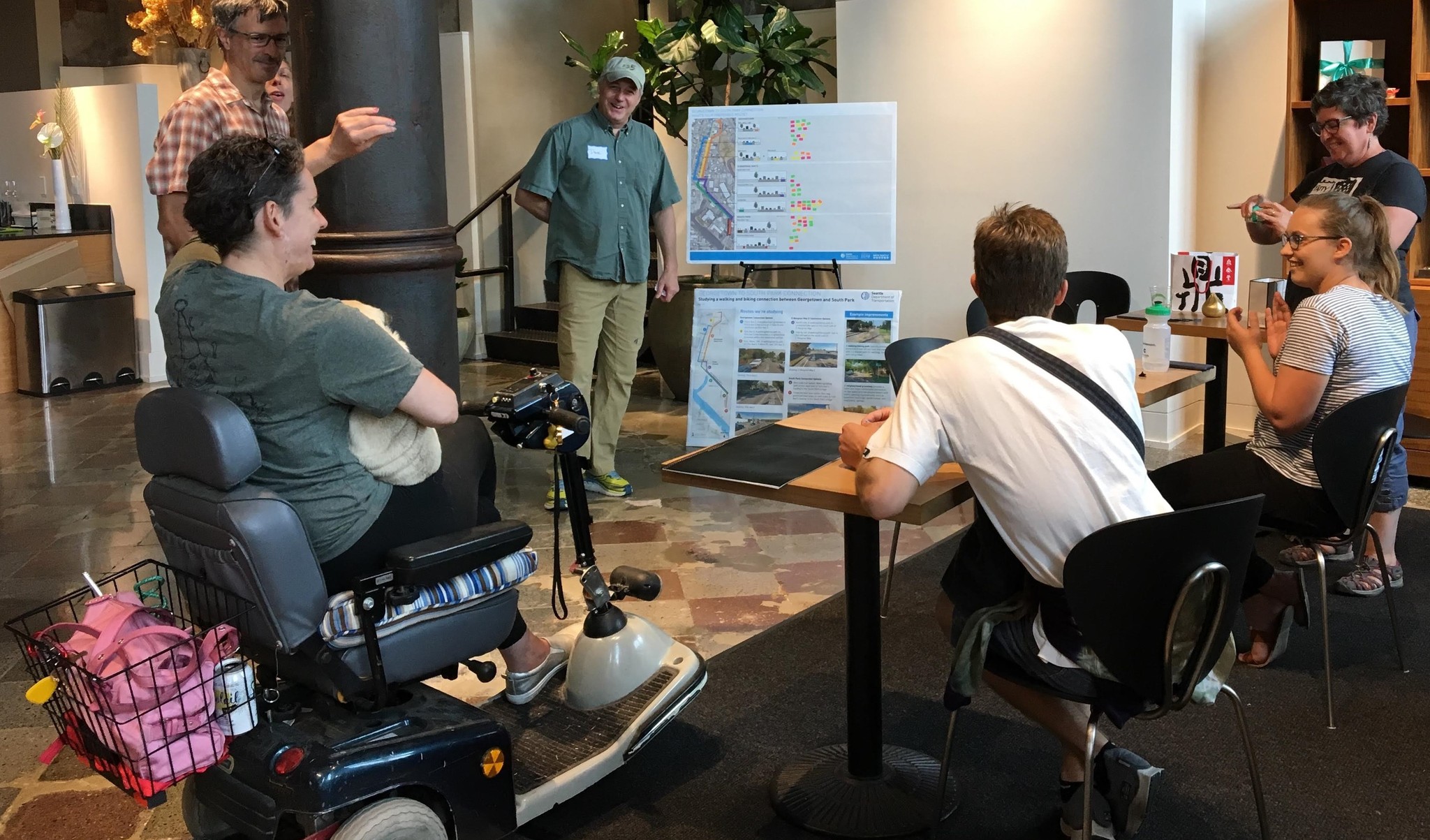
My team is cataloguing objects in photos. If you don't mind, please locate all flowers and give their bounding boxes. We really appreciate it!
[30,74,81,160]
[125,0,220,58]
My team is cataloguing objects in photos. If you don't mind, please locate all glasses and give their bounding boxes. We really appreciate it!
[248,137,286,198]
[222,25,291,47]
[1310,115,1354,136]
[1281,233,1342,251]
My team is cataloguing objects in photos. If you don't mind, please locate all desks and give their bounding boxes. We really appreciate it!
[1104,308,1268,454]
[1132,357,1217,408]
[662,408,973,840]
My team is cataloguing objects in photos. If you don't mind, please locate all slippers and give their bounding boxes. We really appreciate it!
[1238,604,1295,668]
[1289,567,1310,629]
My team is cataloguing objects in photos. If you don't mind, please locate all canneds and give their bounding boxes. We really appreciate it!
[213,657,257,736]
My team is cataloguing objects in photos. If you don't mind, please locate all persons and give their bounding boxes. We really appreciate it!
[1149,190,1417,669]
[515,56,680,512]
[1227,73,1426,597]
[145,0,302,296]
[156,105,586,705]
[839,200,1238,839]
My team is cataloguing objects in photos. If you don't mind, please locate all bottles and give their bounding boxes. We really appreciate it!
[1141,301,1171,373]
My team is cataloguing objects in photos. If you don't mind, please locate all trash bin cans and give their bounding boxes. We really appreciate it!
[13,281,143,397]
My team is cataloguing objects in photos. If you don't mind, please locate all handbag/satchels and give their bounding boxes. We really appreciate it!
[1247,277,1287,329]
[937,512,1040,611]
[34,593,240,783]
[1170,251,1240,314]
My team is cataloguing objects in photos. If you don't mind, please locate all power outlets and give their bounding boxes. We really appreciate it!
[39,176,47,195]
[71,176,79,195]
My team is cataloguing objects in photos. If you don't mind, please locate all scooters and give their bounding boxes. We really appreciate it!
[0,366,708,840]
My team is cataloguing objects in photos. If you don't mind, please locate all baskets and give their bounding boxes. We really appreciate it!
[4,558,288,810]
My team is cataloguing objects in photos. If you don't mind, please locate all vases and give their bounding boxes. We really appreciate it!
[52,158,72,230]
[174,46,212,94]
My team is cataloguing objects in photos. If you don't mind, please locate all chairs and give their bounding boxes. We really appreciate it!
[880,271,1414,840]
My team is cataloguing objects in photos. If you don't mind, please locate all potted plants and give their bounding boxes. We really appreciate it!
[558,0,836,403]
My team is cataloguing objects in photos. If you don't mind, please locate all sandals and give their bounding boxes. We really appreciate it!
[1334,555,1404,597]
[1279,534,1355,566]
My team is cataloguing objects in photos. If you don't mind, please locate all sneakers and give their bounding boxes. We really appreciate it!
[501,621,585,705]
[544,479,568,511]
[1103,747,1167,840]
[1059,783,1116,840]
[583,470,633,497]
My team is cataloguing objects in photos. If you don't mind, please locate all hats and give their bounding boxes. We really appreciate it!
[600,56,646,91]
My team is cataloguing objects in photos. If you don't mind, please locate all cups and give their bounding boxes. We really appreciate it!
[1149,285,1176,312]
